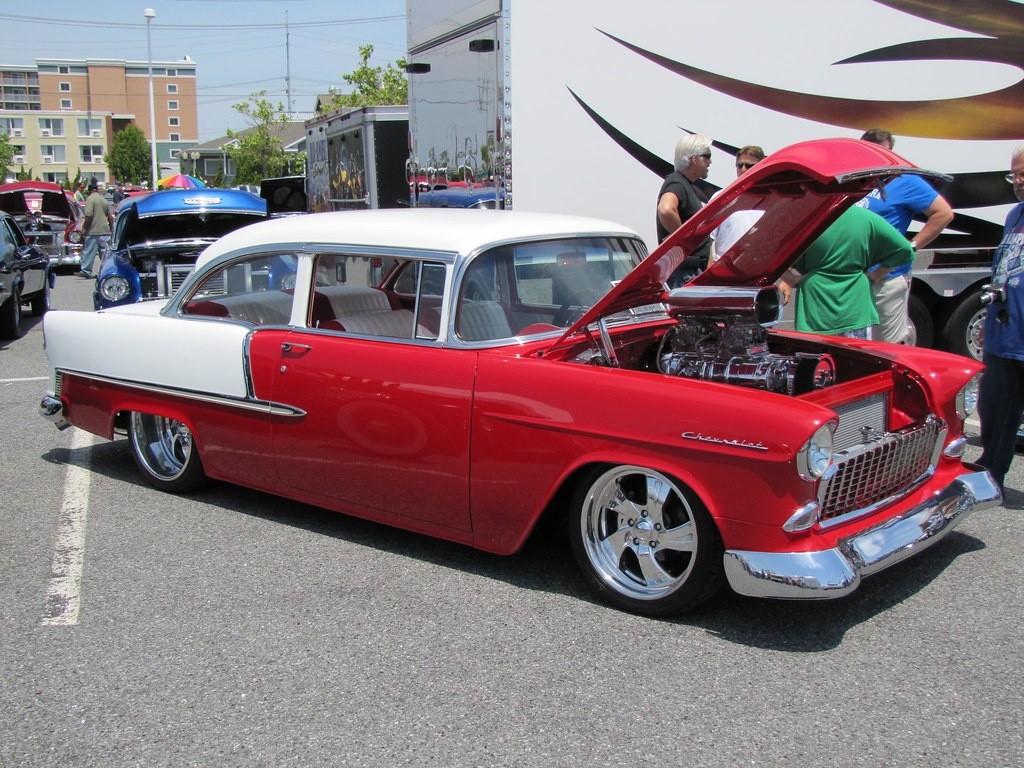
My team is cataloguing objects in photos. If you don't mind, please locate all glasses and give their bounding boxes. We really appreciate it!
[1004,170,1024,184]
[735,162,755,168]
[689,153,712,159]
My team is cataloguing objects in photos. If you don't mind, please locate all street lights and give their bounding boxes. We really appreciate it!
[144,6,159,191]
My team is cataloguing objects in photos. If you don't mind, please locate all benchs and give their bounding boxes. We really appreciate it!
[318,300,520,340]
[187,284,402,324]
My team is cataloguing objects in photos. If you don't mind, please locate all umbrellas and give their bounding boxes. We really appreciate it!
[154,174,206,189]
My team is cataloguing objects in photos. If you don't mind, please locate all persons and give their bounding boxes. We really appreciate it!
[113,184,125,205]
[855,129,955,343]
[776,204,915,338]
[707,146,791,306]
[90,176,98,184]
[74,184,113,278]
[657,135,713,290]
[74,183,85,201]
[974,146,1024,492]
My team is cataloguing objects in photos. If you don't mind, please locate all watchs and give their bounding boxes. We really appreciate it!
[910,239,917,251]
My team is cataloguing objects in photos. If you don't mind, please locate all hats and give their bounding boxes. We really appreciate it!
[77,182,86,188]
[118,184,124,186]
[88,184,98,191]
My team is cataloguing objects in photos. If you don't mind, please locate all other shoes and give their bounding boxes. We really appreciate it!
[74,272,92,279]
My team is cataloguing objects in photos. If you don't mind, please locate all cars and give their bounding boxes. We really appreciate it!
[0,211,57,338]
[36,135,1003,616]
[0,181,83,268]
[91,187,298,312]
